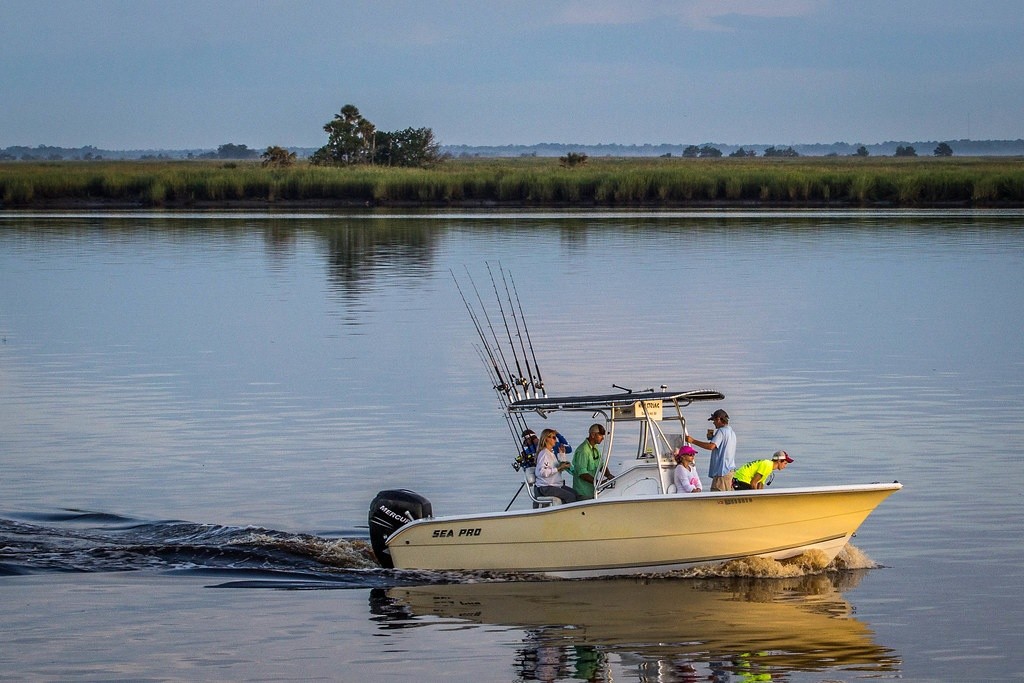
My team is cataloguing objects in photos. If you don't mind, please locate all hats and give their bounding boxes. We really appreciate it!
[708,409,728,421]
[679,446,698,456]
[772,450,794,463]
[589,424,610,435]
[522,434,535,447]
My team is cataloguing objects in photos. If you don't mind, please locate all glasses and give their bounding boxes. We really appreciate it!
[683,454,695,456]
[549,436,556,440]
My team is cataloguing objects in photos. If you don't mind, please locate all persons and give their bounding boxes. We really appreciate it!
[732,450,795,490]
[534,428,579,503]
[674,446,702,493]
[572,423,616,502]
[685,409,737,491]
[520,429,572,509]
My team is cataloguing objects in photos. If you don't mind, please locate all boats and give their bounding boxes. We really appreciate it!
[360,253,907,574]
